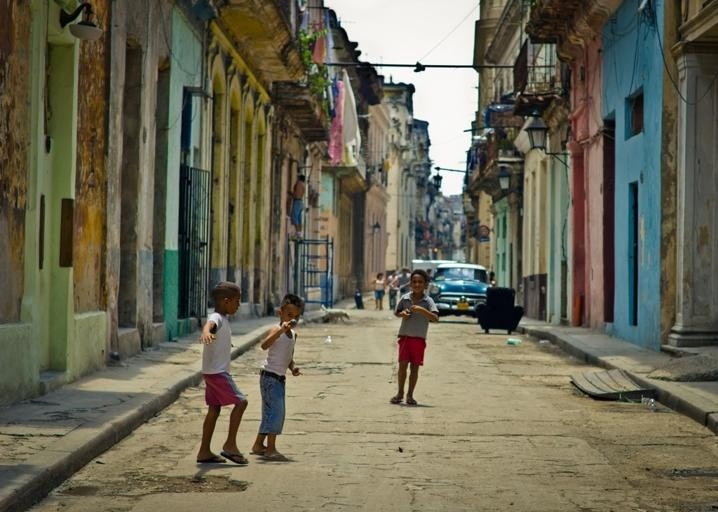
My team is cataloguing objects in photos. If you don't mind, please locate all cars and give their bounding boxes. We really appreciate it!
[427,263,497,324]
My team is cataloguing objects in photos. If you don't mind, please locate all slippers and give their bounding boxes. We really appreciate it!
[391,397,417,405]
[197,447,288,465]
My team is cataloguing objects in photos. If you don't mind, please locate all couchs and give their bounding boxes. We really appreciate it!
[471,285,527,337]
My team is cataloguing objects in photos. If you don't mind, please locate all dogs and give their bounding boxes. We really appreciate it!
[320,304,351,324]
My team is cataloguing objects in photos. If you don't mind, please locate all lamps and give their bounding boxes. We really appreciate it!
[433,166,477,193]
[496,164,523,196]
[57,1,103,42]
[522,105,573,169]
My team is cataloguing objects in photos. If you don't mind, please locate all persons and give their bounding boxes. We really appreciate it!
[487,269,497,286]
[196,281,250,466]
[369,267,434,309]
[249,294,304,462]
[289,174,305,239]
[388,271,439,404]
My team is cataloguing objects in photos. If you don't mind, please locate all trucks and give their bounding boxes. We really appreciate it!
[411,258,458,282]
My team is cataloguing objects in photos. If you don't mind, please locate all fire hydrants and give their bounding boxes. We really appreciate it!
[352,289,364,309]
